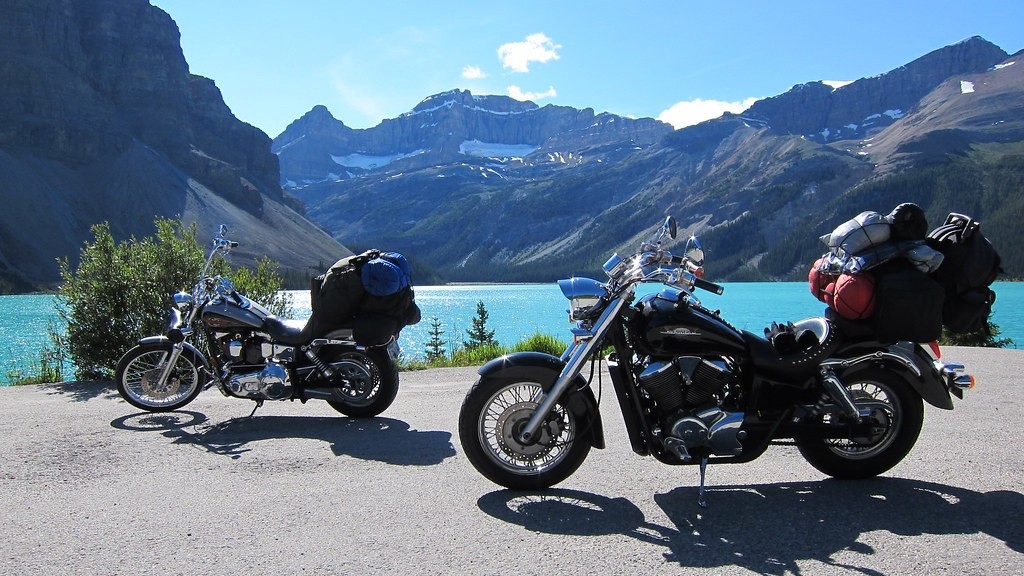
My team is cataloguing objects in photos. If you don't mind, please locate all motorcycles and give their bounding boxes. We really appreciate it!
[457,216,975,508]
[115,223,404,419]
